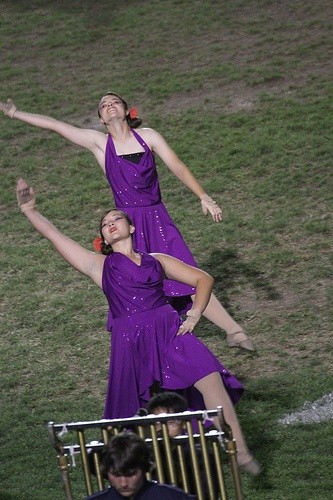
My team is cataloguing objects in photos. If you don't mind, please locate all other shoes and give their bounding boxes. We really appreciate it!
[229,453,262,475]
[226,331,256,351]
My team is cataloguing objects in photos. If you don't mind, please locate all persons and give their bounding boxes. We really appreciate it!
[16,177,265,481]
[82,431,199,500]
[87,391,224,500]
[0,91,260,358]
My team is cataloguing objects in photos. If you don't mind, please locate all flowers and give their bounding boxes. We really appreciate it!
[130,107,137,118]
[93,237,102,252]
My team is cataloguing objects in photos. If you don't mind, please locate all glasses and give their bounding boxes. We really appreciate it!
[153,419,179,430]
